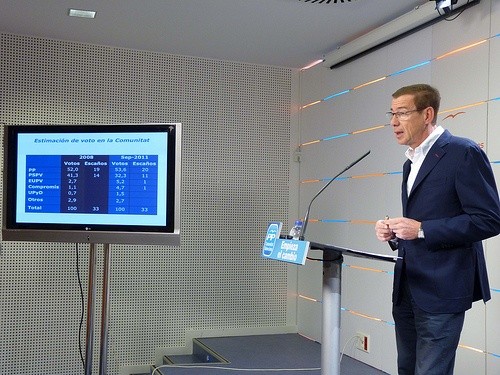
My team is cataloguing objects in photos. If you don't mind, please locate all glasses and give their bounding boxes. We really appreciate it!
[385,109,422,120]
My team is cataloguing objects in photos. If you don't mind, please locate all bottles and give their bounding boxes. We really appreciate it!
[289,220,303,240]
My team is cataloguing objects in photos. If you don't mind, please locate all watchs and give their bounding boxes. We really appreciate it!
[417,224,426,239]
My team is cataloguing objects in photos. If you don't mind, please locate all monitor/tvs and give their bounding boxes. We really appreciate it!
[0,122,183,247]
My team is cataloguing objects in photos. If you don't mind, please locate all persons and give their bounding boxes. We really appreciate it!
[372,82,500,375]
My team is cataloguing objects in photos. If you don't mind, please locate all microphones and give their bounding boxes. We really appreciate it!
[298,150,372,241]
